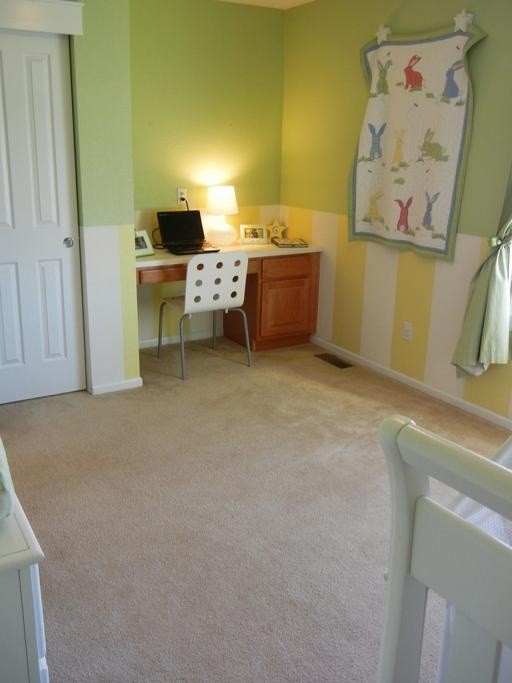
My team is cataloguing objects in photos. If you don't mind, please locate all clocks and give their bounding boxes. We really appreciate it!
[267,219,286,240]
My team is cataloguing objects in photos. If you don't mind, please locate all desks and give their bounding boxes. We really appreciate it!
[134,241,323,352]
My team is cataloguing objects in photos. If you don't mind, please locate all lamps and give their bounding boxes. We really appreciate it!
[204,184,240,247]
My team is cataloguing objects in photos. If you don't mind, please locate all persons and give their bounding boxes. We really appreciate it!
[251,229,258,238]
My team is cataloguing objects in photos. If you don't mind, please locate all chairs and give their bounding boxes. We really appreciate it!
[375,413,511,681]
[153,251,253,379]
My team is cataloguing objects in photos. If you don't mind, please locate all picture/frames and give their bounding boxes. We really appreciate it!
[135,228,155,258]
[240,224,268,244]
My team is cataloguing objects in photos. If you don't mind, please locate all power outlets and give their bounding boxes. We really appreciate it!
[177,188,187,205]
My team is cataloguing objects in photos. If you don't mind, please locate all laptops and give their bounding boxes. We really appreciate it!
[156,210,220,255]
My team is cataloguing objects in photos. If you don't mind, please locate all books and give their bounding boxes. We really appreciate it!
[271,237,308,248]
